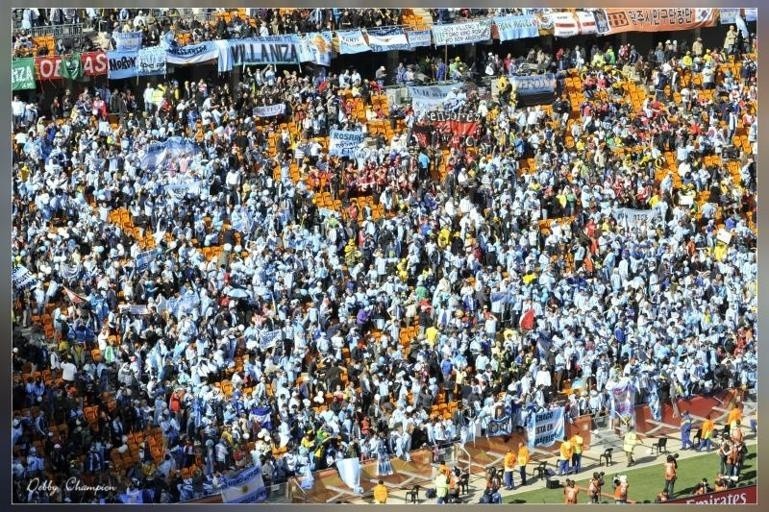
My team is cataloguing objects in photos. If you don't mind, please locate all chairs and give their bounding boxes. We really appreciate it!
[13,6,761,505]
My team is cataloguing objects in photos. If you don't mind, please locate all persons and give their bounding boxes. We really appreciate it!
[10,6,756,504]
